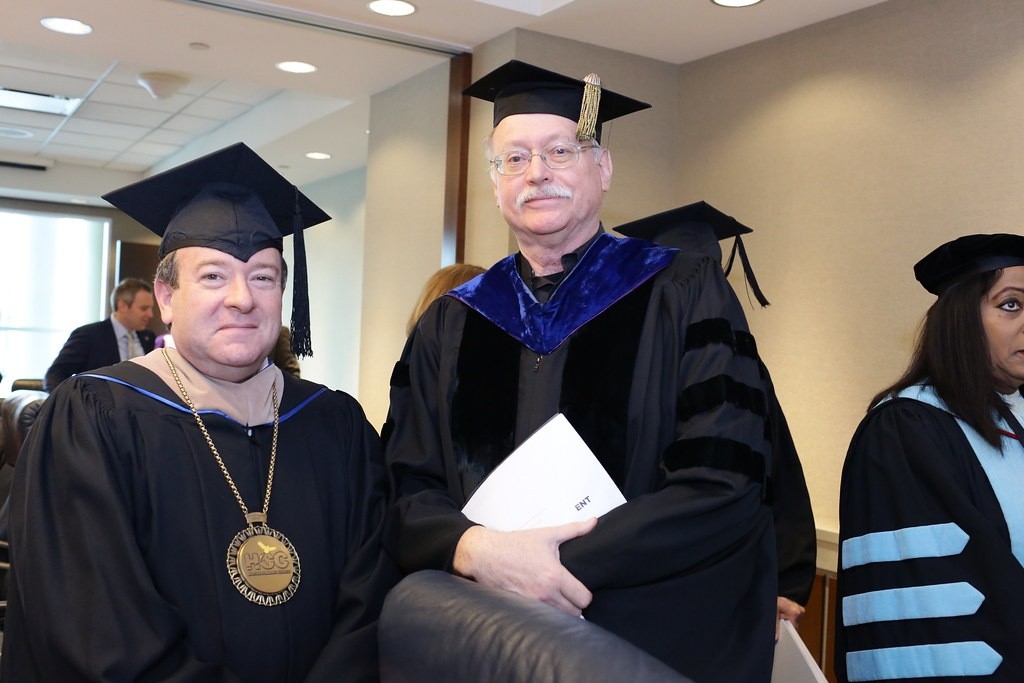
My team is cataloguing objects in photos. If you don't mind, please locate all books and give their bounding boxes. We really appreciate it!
[772,617,829,683]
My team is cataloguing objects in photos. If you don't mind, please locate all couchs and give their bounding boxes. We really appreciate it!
[0,390,48,620]
[377,570,693,683]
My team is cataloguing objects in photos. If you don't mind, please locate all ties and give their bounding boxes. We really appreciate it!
[123,334,137,359]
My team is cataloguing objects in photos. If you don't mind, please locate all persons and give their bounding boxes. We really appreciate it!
[384,59,819,683]
[0,139,383,683]
[833,232,1024,683]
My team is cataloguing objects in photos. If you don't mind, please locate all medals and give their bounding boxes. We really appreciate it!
[226,525,302,607]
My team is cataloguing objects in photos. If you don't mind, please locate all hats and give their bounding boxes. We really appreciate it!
[612,201,771,311]
[461,60,652,143]
[102,141,333,360]
[913,232,1024,296]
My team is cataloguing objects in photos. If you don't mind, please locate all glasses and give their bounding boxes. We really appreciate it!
[488,143,600,175]
